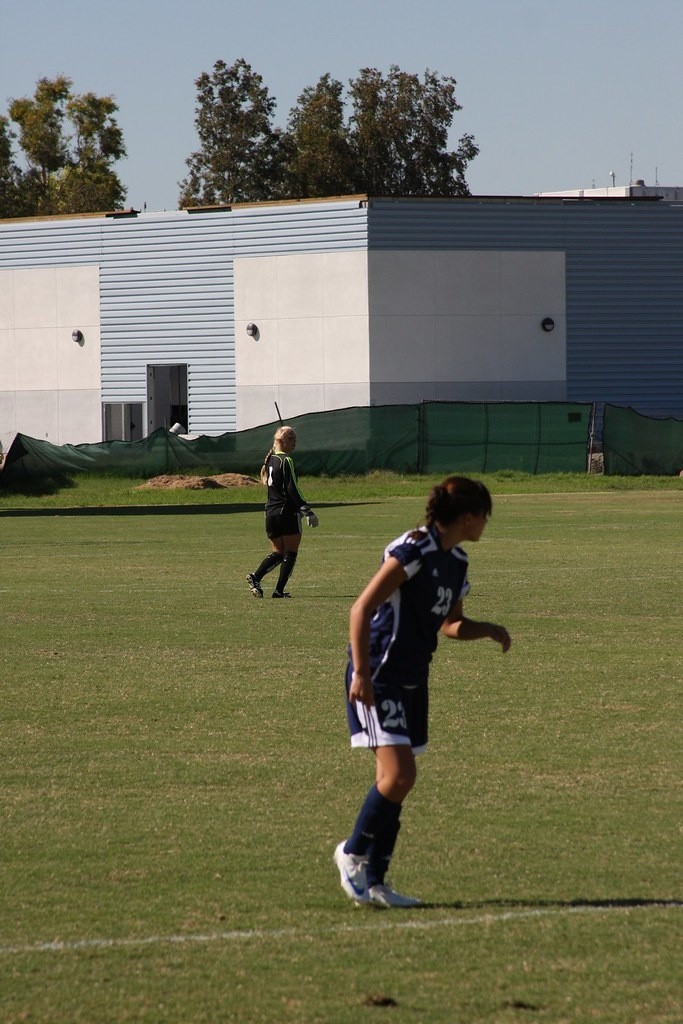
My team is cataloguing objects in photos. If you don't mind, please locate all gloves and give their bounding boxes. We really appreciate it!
[306,514,319,527]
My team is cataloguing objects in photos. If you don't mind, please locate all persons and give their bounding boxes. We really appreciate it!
[246,426,319,598]
[334,477,511,906]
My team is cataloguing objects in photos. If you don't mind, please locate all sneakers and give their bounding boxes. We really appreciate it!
[246,573,264,598]
[333,839,370,904]
[271,589,291,598]
[366,885,421,908]
